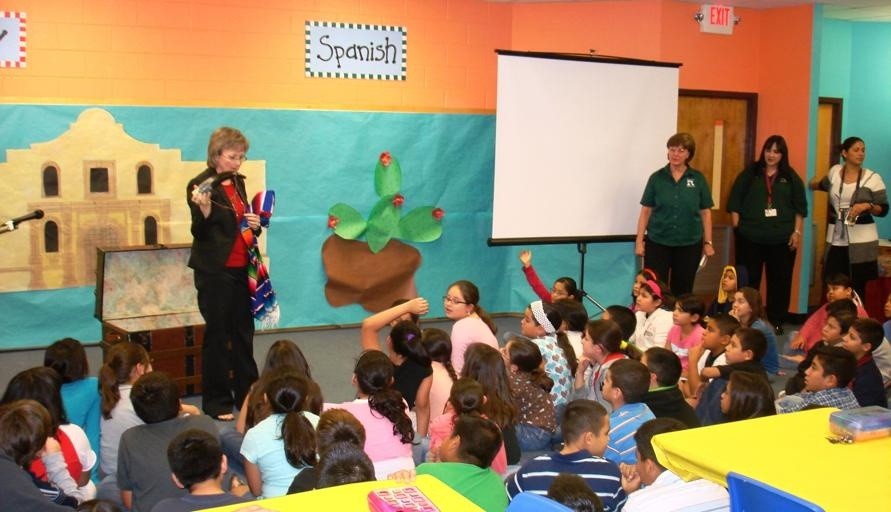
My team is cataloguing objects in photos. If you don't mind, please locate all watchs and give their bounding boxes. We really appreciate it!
[702,241,712,245]
[868,201,874,211]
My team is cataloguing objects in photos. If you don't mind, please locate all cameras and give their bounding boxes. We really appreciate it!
[843,212,859,226]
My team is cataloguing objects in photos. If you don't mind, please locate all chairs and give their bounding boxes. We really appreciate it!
[504,492,574,511]
[721,466,828,512]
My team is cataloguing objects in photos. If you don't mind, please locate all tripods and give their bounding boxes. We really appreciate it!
[576,243,607,311]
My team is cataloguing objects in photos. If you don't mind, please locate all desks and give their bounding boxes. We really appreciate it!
[178,462,491,512]
[650,408,891,512]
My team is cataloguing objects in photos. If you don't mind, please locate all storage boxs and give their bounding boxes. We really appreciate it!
[93,241,210,404]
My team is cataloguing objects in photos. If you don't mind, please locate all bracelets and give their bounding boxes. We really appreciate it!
[793,228,803,238]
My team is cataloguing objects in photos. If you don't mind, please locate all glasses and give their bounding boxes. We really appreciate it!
[550,288,568,296]
[442,294,468,307]
[221,152,247,162]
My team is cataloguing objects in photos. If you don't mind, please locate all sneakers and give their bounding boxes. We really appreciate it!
[772,320,786,335]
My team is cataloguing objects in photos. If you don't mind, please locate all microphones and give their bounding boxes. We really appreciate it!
[11,209,44,223]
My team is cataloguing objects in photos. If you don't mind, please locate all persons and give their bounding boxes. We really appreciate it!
[183,124,277,425]
[631,129,716,314]
[807,136,890,306]
[725,134,808,336]
